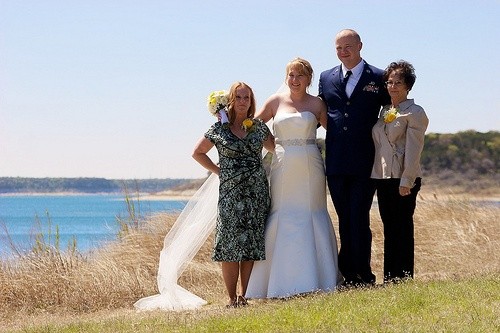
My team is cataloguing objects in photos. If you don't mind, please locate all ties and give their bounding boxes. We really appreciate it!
[342,71,352,89]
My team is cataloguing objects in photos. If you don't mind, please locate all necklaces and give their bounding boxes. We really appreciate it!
[290,93,305,111]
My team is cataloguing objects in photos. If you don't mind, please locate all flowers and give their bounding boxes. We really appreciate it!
[240,118,255,132]
[208,90,230,128]
[383,104,398,123]
[363,81,380,94]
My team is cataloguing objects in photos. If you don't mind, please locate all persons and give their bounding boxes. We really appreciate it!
[214,56,344,301]
[315,28,391,291]
[369,59,430,285]
[191,82,323,308]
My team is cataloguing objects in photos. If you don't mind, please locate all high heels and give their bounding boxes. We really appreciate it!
[237,296,248,308]
[226,295,237,309]
